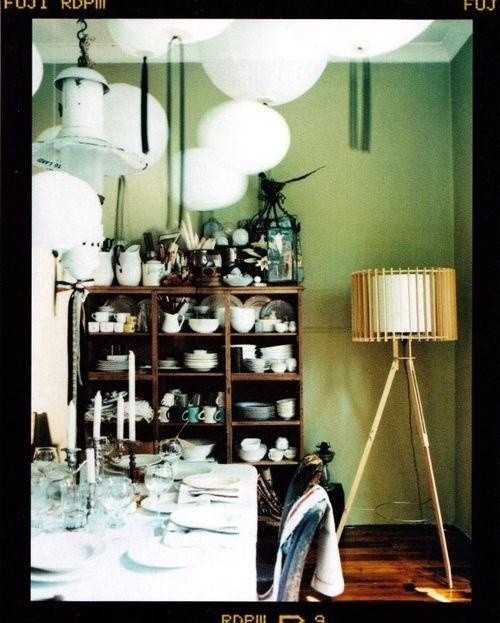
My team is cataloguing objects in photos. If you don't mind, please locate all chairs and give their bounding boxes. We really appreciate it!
[257,449,331,601]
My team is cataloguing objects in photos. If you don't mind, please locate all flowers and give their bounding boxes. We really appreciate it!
[240,233,272,272]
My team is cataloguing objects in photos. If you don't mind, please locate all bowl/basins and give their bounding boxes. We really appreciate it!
[277,398,296,420]
[160,360,175,366]
[241,438,262,450]
[230,306,256,333]
[238,444,268,461]
[187,316,221,333]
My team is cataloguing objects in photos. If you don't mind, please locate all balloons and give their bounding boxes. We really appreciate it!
[166,147,249,209]
[101,80,166,166]
[33,169,101,251]
[108,17,437,105]
[33,43,44,94]
[198,97,290,172]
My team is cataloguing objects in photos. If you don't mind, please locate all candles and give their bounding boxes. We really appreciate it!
[129,348,136,443]
[92,389,102,439]
[116,393,124,440]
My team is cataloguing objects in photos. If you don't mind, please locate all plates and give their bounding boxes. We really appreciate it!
[104,295,137,315]
[96,359,128,372]
[200,295,244,331]
[160,367,181,372]
[183,350,221,372]
[220,341,298,372]
[260,299,297,333]
[243,295,271,320]
[234,402,276,420]
[30,438,242,575]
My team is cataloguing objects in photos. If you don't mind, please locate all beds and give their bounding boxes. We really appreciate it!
[31,456,261,602]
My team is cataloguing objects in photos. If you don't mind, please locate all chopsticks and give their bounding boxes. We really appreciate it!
[157,294,187,315]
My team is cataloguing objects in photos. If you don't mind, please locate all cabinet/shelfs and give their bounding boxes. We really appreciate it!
[70,283,306,465]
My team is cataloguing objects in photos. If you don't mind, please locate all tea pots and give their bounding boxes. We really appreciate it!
[161,311,184,333]
[142,259,173,286]
[114,241,142,286]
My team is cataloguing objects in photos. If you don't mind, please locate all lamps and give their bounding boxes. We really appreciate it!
[32,18,149,208]
[316,263,462,587]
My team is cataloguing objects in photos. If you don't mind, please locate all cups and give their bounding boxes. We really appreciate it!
[157,390,225,422]
[268,436,297,462]
[89,305,130,323]
[89,323,135,333]
